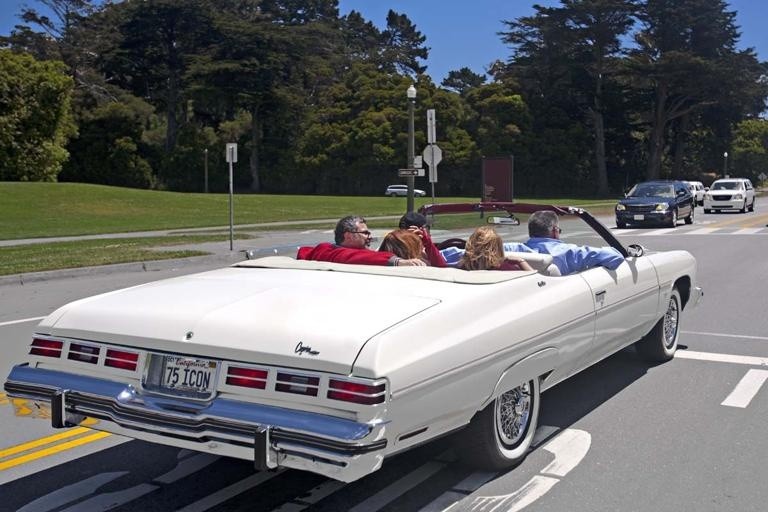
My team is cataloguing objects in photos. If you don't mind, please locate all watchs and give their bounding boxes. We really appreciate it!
[518,258,525,263]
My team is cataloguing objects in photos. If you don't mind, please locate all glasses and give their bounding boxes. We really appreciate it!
[351,230,371,237]
[555,226,562,234]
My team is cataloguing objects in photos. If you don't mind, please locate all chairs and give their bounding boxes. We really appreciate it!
[506,250,560,279]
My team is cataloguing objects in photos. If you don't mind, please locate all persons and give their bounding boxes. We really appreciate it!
[377,224,450,268]
[296,215,428,268]
[397,210,465,263]
[501,208,626,277]
[453,225,536,272]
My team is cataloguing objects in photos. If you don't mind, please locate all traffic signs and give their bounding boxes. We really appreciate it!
[399,169,419,176]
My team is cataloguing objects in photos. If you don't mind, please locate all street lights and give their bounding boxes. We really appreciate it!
[724,151,728,178]
[407,85,417,210]
[204,148,208,193]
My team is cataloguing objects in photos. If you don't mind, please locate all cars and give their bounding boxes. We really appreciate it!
[615,178,755,228]
[4,201,704,483]
[385,184,426,197]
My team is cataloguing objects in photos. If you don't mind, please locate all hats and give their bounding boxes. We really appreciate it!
[398,210,435,230]
